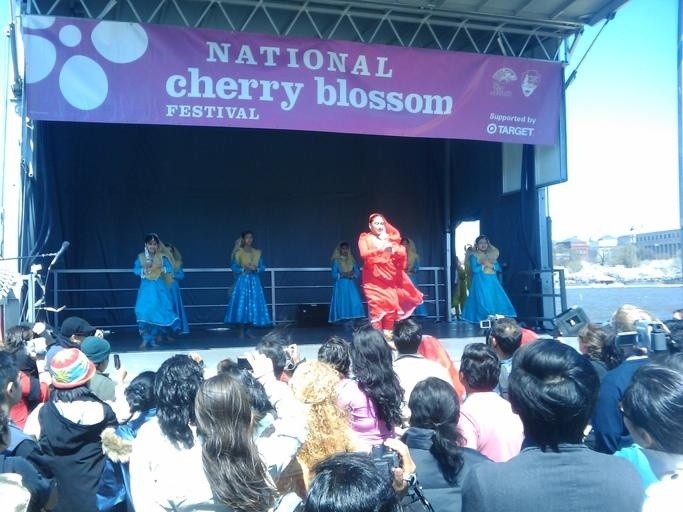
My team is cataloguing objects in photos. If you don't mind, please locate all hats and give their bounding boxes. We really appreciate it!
[50,347,96,388]
[81,336,111,363]
[61,317,96,337]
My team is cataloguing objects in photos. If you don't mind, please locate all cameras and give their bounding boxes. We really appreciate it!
[237,357,253,370]
[98,329,111,339]
[283,349,296,370]
[362,446,404,483]
[616,322,671,352]
[478,315,503,329]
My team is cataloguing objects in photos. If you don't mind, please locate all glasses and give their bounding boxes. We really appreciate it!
[616,402,631,421]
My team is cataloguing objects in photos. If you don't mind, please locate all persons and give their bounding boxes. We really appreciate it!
[335,326,407,453]
[463,244,475,294]
[157,243,190,344]
[129,351,202,512]
[9,370,53,430]
[638,317,683,373]
[94,371,159,512]
[591,303,661,454]
[327,241,367,332]
[459,235,517,326]
[418,334,466,404]
[485,322,522,401]
[611,353,682,490]
[0,351,57,512]
[0,410,52,512]
[578,322,624,384]
[256,341,289,384]
[617,363,683,512]
[80,335,118,401]
[358,213,423,349]
[451,256,467,321]
[402,238,428,318]
[296,438,416,512]
[290,362,378,500]
[317,334,350,378]
[216,360,277,438]
[43,316,103,372]
[390,317,456,427]
[23,346,134,441]
[497,317,541,347]
[396,377,494,512]
[38,348,120,512]
[0,326,40,379]
[461,339,645,512]
[454,342,526,463]
[148,351,309,512]
[223,230,272,340]
[133,232,182,349]
[257,344,306,384]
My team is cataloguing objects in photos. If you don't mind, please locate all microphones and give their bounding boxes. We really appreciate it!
[49,240,71,273]
